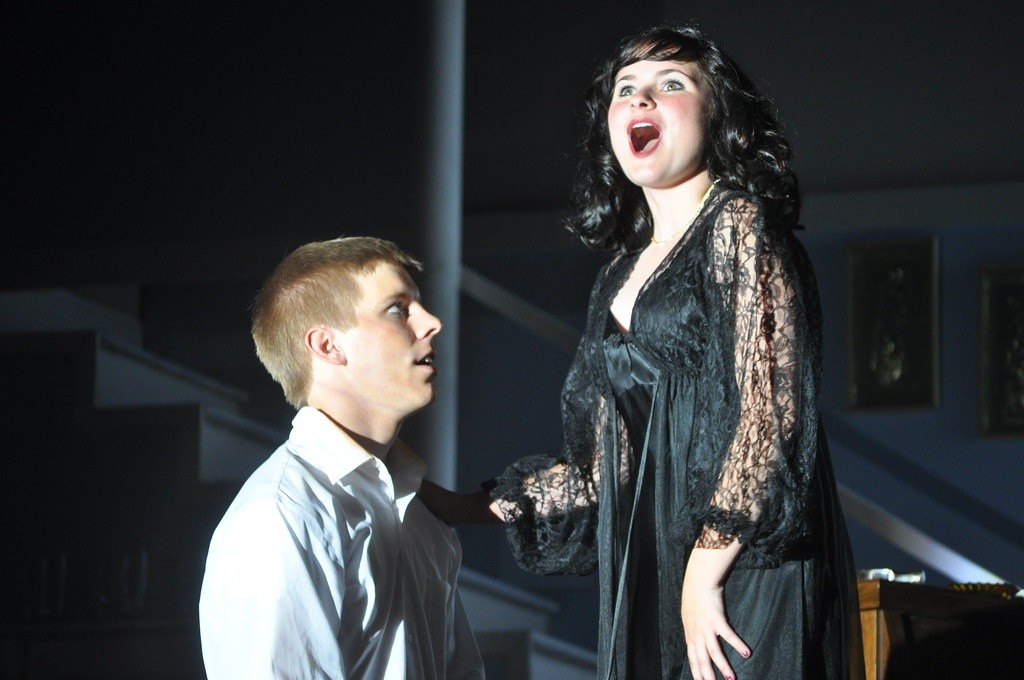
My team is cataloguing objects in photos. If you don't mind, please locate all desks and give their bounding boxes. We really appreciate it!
[857,579,1024,680]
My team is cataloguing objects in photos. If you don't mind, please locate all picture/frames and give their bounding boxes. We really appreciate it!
[843,236,944,416]
[976,260,1024,440]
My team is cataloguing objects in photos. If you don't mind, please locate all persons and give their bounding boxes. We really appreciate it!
[198,236,487,680]
[418,28,865,680]
